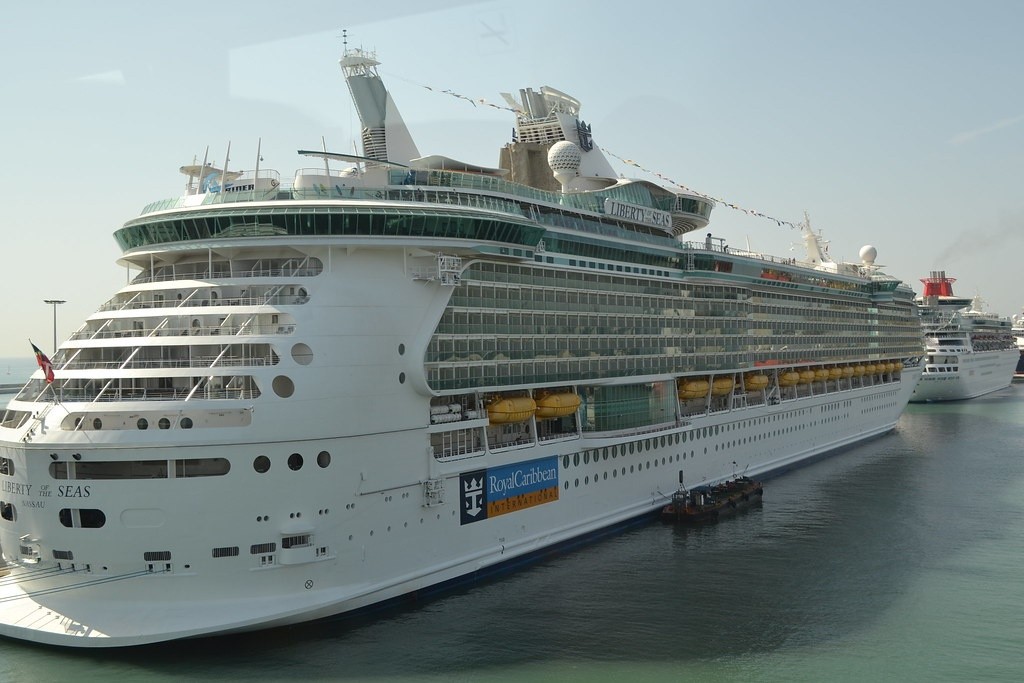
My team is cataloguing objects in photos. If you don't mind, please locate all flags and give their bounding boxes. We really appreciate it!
[29,339,54,383]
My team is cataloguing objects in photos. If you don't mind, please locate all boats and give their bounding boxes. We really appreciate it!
[679,379,710,399]
[865,364,876,376]
[779,371,799,386]
[886,363,895,373]
[536,391,582,421]
[895,362,904,372]
[658,460,765,523]
[745,374,769,391]
[828,367,842,380]
[486,395,537,428]
[798,370,815,384]
[854,364,866,377]
[876,364,886,375]
[842,366,854,378]
[814,369,830,381]
[711,377,733,397]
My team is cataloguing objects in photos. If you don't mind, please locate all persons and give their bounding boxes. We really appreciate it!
[706,233,713,250]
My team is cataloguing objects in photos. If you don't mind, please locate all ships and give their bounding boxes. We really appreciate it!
[1,27,929,652]
[908,269,1024,402]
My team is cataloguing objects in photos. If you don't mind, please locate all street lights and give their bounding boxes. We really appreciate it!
[43,299,68,353]
